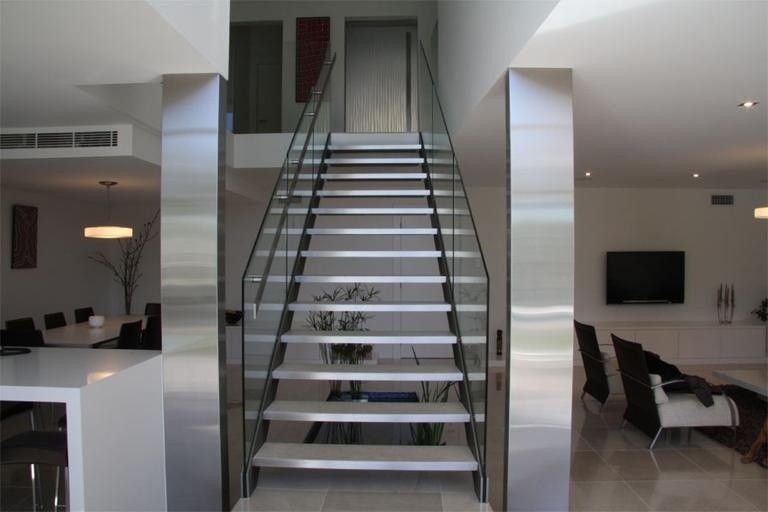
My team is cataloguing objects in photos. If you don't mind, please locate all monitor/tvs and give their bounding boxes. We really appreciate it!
[606,251,685,306]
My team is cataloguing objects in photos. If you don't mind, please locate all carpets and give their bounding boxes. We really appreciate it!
[327,388,420,402]
[690,383,768,471]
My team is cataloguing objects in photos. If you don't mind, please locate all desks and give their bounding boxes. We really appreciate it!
[41,314,149,347]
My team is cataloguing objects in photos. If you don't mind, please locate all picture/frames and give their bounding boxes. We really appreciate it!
[10,204,38,269]
[295,16,331,105]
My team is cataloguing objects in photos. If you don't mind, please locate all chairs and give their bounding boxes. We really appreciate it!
[574,316,740,453]
[118,300,161,348]
[0,304,95,347]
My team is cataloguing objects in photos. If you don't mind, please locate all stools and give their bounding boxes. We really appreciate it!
[0,428,68,512]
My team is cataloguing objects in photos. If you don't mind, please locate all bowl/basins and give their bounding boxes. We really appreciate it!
[89,314,104,328]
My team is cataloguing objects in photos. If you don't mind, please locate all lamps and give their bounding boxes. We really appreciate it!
[81,179,134,240]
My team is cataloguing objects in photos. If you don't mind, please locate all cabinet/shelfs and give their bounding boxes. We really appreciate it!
[574,321,767,367]
